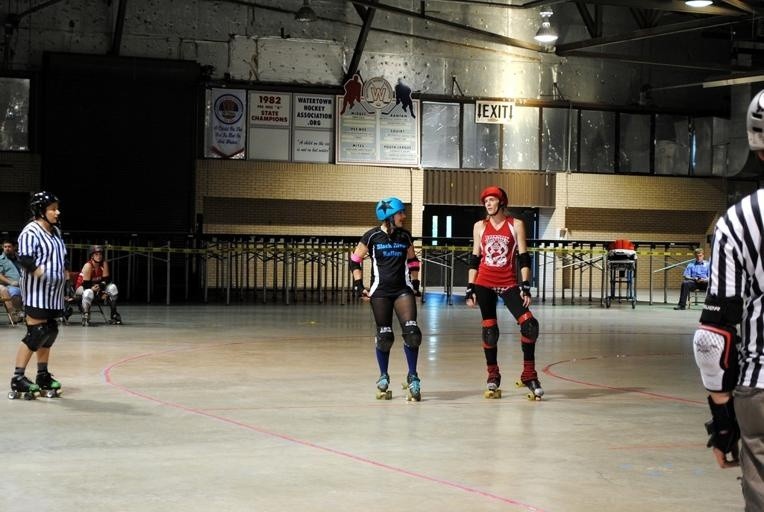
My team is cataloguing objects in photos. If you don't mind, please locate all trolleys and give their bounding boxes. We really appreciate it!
[602,257,637,310]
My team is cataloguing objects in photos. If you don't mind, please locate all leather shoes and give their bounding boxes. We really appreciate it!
[673,305,685,310]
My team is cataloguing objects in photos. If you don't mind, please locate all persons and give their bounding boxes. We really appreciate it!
[463,186,545,397]
[690,89,764,512]
[0,240,26,318]
[673,247,709,310]
[348,197,421,398]
[73,246,121,322]
[8,188,68,391]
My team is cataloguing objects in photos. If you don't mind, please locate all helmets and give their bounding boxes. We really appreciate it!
[89,245,103,255]
[376,198,405,221]
[746,90,764,152]
[29,190,61,217]
[480,185,508,206]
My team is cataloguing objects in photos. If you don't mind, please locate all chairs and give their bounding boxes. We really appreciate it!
[680,268,709,309]
[0,272,109,327]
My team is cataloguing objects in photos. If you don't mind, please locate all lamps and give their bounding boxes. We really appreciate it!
[533,18,559,44]
[539,4,554,16]
[294,0,317,22]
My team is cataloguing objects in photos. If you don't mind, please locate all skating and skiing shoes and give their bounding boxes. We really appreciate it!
[515,370,544,401]
[376,373,392,399]
[8,373,64,400]
[82,312,91,326]
[400,372,421,401]
[109,311,121,325]
[485,372,501,399]
